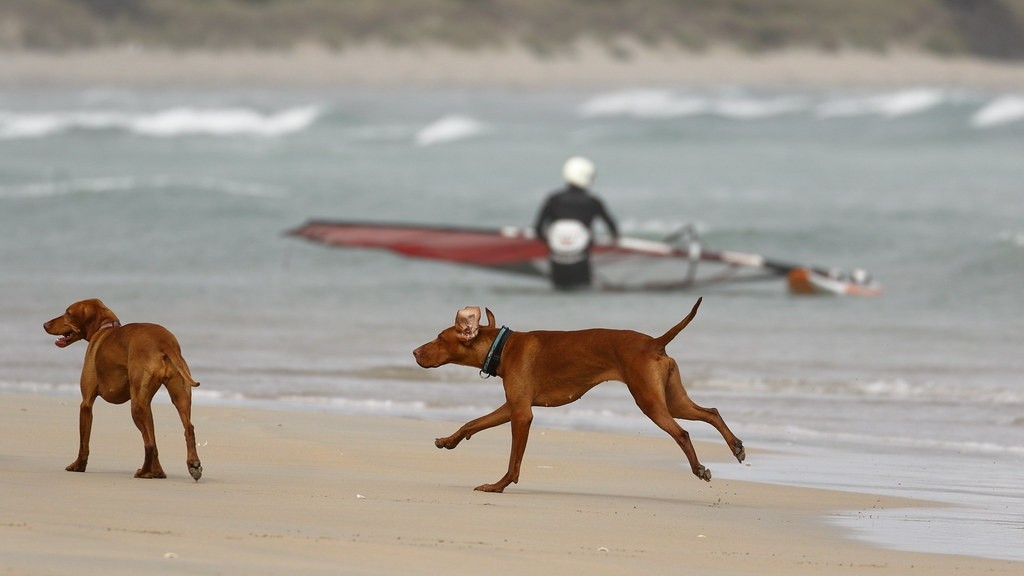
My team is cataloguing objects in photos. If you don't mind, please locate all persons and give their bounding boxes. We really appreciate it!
[534,155,619,294]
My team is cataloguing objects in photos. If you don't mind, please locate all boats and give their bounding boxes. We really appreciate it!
[281,218,878,302]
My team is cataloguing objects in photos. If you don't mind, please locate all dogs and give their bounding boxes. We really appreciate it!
[414,296,746,493]
[42,298,204,482]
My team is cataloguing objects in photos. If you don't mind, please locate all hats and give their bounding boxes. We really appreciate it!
[564,156,596,189]
